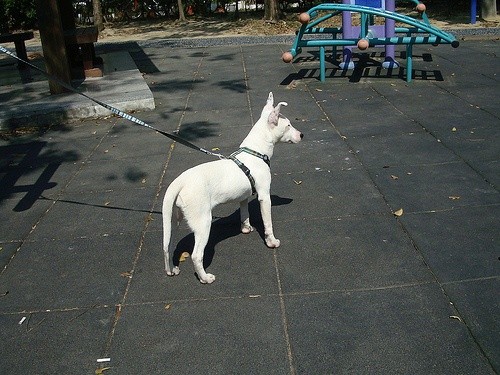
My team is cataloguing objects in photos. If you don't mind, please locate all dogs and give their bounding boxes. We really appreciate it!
[161,92,305,283]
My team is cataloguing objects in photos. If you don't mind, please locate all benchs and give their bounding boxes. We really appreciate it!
[76,27,103,79]
[0,32,34,67]
